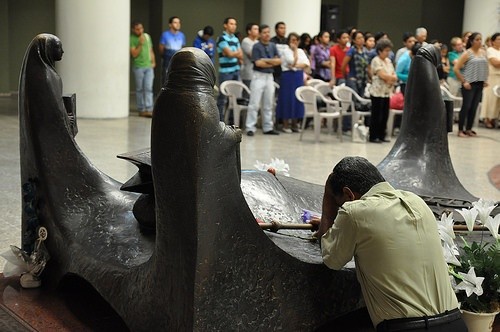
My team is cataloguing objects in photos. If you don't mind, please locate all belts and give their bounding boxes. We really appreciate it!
[381,311,462,329]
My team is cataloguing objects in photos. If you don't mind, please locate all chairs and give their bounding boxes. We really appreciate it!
[220,78,500,143]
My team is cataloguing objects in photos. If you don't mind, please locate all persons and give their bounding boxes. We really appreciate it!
[21,33,83,257]
[151,46,243,259]
[216,18,500,144]
[131,21,156,117]
[192,25,215,65]
[309,155,470,332]
[159,17,187,87]
[375,44,469,196]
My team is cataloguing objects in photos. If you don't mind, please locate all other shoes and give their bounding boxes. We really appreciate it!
[292,128,301,132]
[281,127,292,133]
[373,139,380,142]
[146,111,153,117]
[264,130,279,135]
[485,122,493,129]
[382,138,390,142]
[247,131,252,135]
[135,110,148,117]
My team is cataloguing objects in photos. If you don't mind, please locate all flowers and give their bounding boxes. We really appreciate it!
[435,197,500,312]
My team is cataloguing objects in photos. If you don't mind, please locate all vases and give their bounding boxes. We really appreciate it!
[458,302,500,332]
